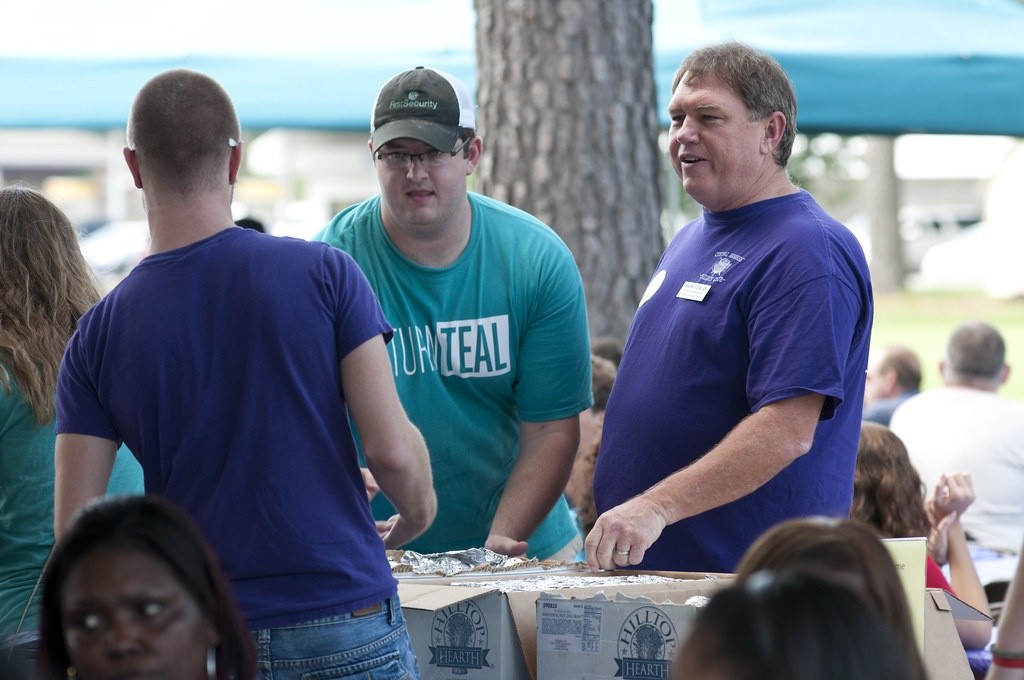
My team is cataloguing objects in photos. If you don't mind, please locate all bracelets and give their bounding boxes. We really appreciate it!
[989,644,1024,667]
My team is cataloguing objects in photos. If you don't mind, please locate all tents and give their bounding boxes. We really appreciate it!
[0,0,1024,137]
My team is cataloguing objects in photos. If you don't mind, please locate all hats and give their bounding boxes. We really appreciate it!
[370,65,477,154]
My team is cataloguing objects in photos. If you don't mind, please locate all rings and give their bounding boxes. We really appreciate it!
[615,549,629,555]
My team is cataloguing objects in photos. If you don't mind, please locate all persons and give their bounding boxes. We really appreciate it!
[38,494,256,680]
[985,540,1024,680]
[678,570,909,680]
[890,322,1024,625]
[740,518,928,680]
[564,339,624,563]
[313,67,594,560]
[55,70,438,679]
[584,43,873,573]
[862,346,922,426]
[0,188,145,680]
[850,422,997,676]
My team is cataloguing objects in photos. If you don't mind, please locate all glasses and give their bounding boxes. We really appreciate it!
[378,137,471,171]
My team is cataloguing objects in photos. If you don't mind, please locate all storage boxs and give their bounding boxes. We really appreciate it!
[386,548,996,680]
